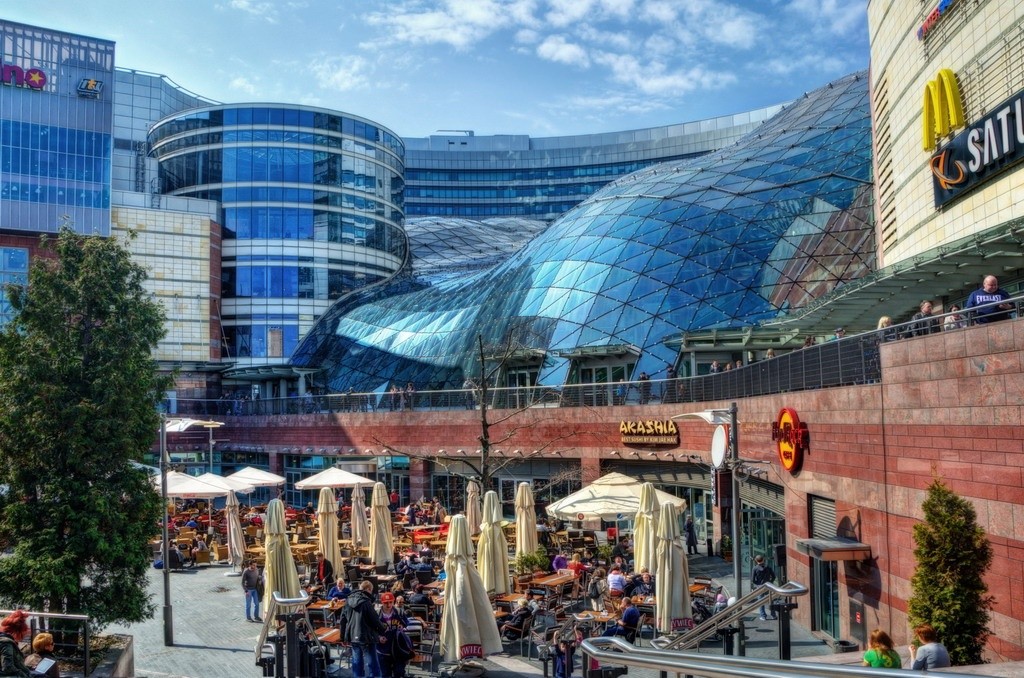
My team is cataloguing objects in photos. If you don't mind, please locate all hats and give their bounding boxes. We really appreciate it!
[381,592,394,602]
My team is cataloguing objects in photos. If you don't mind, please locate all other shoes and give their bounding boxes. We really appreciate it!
[759,616,766,620]
[771,616,777,620]
[247,618,254,622]
[254,617,263,623]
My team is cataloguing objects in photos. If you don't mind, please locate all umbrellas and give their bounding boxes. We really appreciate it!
[143,463,700,678]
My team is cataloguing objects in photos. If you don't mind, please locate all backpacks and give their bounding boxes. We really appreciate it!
[588,577,604,599]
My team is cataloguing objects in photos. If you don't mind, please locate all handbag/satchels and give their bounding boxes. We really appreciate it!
[394,630,416,660]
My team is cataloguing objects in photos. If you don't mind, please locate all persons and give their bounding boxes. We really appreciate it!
[723,363,733,371]
[0,610,42,678]
[966,274,1016,325]
[804,335,816,347]
[617,378,628,405]
[943,304,968,331]
[910,298,941,336]
[639,372,652,404]
[736,360,742,367]
[908,624,952,671]
[32,632,60,678]
[765,348,776,359]
[461,376,478,410]
[713,594,726,614]
[752,555,778,621]
[862,628,903,669]
[828,327,847,341]
[709,359,723,374]
[877,315,901,341]
[213,381,420,419]
[665,365,678,402]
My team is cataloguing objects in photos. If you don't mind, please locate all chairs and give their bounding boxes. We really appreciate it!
[159,498,727,678]
